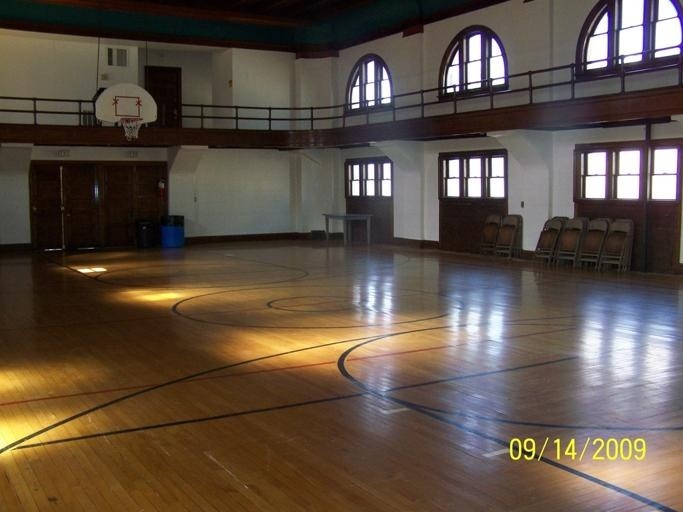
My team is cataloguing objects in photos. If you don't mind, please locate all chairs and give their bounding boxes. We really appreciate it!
[478,212,635,273]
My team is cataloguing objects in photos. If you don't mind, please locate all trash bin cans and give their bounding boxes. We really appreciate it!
[136,215,185,250]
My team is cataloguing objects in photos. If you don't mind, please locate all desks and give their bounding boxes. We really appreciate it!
[321,213,374,247]
